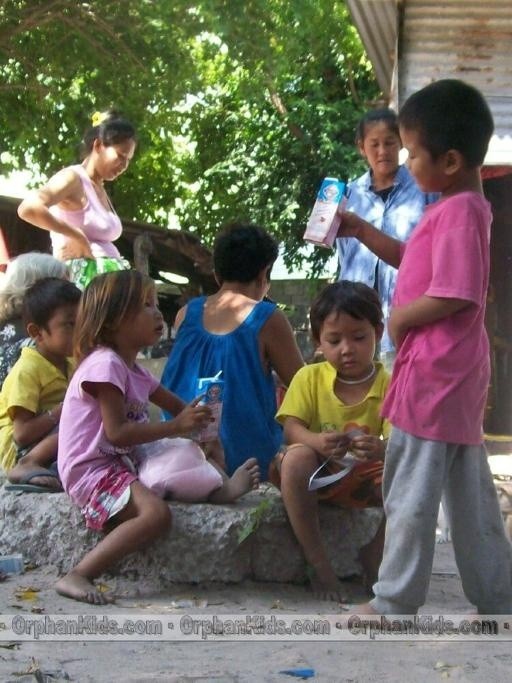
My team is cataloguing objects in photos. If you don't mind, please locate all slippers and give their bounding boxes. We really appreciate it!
[4,467,63,494]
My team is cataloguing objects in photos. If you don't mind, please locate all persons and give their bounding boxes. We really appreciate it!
[18,108,139,292]
[335,105,439,374]
[273,278,394,604]
[329,76,510,632]
[158,223,307,492]
[0,276,84,495]
[51,268,263,606]
[0,249,74,388]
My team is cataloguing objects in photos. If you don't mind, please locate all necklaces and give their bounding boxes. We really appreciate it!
[334,362,377,385]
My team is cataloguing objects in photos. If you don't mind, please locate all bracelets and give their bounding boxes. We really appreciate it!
[46,407,60,428]
